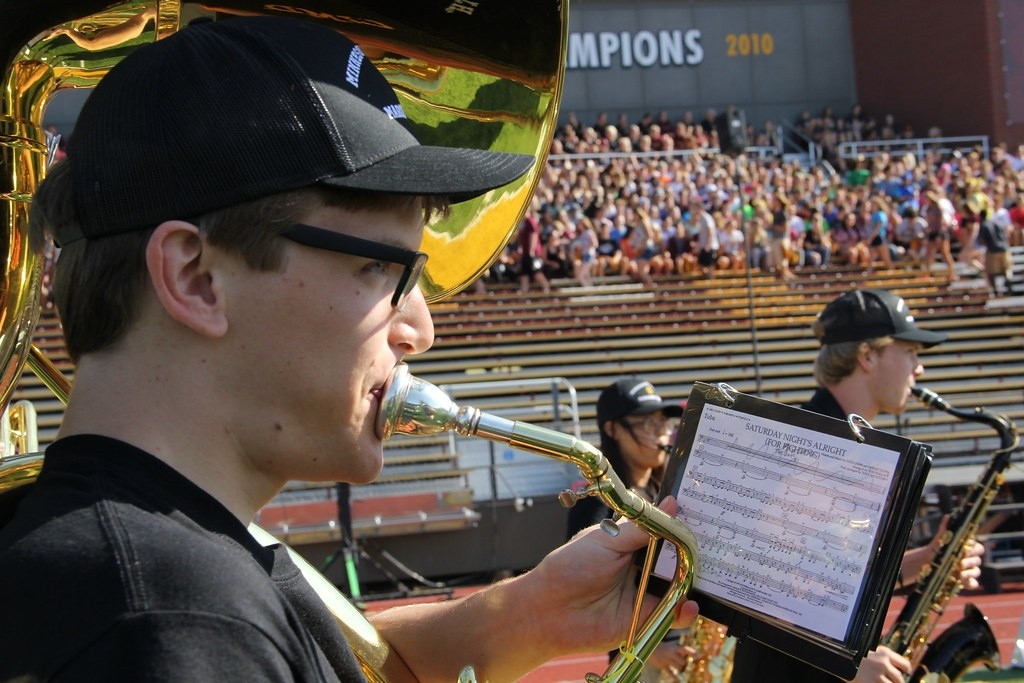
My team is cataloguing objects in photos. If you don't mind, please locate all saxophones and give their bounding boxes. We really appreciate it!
[879,386,1018,683]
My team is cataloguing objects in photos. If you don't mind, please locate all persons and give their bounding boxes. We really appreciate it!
[564,380,684,663]
[0,13,697,683]
[730,288,984,683]
[482,105,1024,294]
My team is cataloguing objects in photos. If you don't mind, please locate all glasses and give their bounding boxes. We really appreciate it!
[623,413,675,432]
[275,222,429,310]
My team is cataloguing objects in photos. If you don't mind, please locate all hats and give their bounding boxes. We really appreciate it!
[812,285,948,343]
[595,377,683,432]
[32,9,537,250]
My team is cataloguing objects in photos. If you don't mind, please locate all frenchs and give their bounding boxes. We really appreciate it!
[0,0,698,683]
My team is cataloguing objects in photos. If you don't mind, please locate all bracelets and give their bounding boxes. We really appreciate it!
[897,571,907,591]
